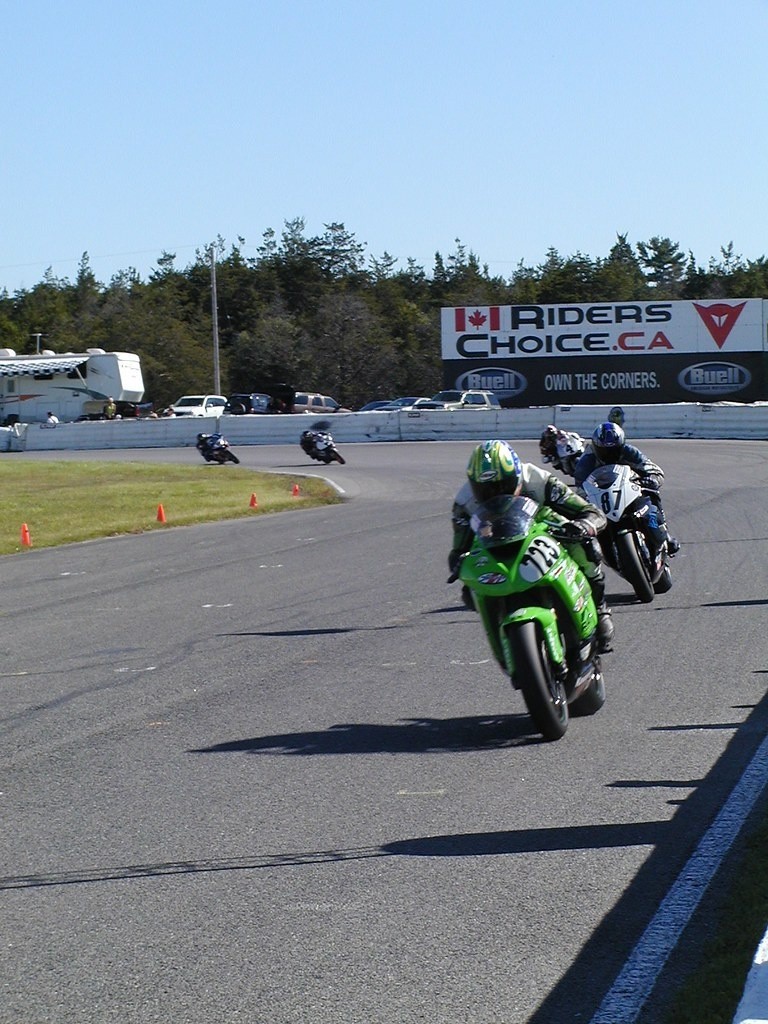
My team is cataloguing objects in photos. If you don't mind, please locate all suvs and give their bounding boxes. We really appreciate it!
[164,395,227,418]
[413,389,502,411]
[223,393,275,415]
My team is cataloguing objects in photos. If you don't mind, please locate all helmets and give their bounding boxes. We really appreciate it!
[591,422,626,466]
[465,440,524,513]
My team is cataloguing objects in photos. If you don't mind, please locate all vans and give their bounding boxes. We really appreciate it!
[273,383,353,413]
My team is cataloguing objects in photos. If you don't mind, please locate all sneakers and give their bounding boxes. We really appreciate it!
[593,603,615,644]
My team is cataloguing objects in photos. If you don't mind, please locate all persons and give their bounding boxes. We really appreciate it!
[447,441,616,643]
[573,422,681,568]
[104,397,123,419]
[196,432,229,463]
[147,408,176,418]
[538,425,584,469]
[300,431,332,461]
[47,412,59,423]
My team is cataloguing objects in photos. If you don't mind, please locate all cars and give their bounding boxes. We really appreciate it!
[359,396,432,411]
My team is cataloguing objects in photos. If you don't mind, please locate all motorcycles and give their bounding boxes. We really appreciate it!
[202,436,240,464]
[307,433,346,465]
[541,433,585,476]
[575,463,673,603]
[448,494,613,739]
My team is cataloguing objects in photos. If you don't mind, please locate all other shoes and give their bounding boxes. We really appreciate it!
[667,538,683,555]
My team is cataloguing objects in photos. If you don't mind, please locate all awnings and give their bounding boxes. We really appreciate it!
[0,356,91,377]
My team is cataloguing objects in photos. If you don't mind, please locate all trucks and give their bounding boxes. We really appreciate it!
[0,347,145,422]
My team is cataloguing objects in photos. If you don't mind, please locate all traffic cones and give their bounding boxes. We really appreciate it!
[20,524,32,546]
[292,484,299,495]
[248,493,258,507]
[155,504,166,522]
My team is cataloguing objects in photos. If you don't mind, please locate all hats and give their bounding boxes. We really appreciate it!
[167,409,174,412]
[47,411,53,415]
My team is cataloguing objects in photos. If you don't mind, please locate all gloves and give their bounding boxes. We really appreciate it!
[561,521,589,544]
[447,549,460,572]
[639,476,661,491]
